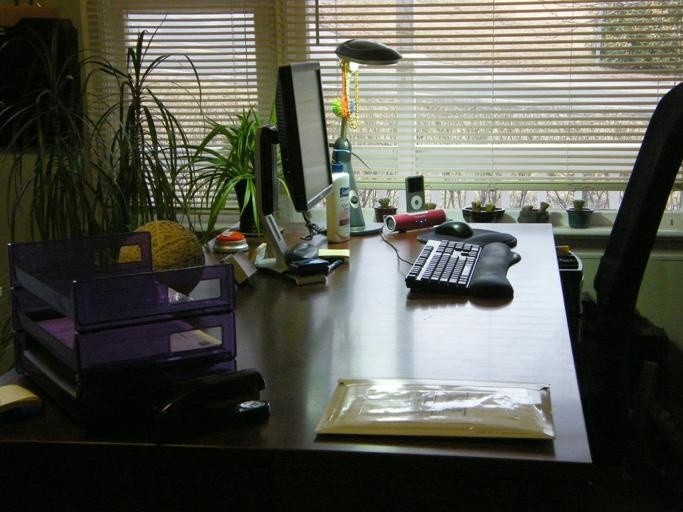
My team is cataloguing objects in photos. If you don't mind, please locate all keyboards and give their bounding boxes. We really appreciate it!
[403,240,482,293]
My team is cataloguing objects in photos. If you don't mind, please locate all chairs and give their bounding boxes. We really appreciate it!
[565,79,681,342]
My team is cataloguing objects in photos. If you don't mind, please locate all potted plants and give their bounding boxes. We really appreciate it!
[517,202,548,223]
[461,201,504,223]
[568,197,591,227]
[180,99,287,238]
[374,198,395,223]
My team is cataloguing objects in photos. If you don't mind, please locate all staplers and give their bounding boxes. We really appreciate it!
[147,368,270,434]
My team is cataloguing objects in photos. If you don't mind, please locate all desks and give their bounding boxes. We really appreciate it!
[0,223,595,469]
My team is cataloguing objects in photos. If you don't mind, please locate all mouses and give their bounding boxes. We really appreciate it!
[434,221,473,238]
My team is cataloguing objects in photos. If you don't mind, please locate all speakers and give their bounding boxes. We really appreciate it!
[384,208,446,233]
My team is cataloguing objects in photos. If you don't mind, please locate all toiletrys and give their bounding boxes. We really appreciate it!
[325,161,351,244]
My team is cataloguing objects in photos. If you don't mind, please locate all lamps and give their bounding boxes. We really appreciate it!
[331,39,404,228]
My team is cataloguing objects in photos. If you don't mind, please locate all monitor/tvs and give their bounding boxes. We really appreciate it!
[254,61,344,287]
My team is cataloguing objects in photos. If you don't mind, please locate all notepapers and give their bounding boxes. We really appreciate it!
[318,248,351,259]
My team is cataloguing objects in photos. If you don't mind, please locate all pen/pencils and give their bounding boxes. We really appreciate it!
[330,259,342,270]
